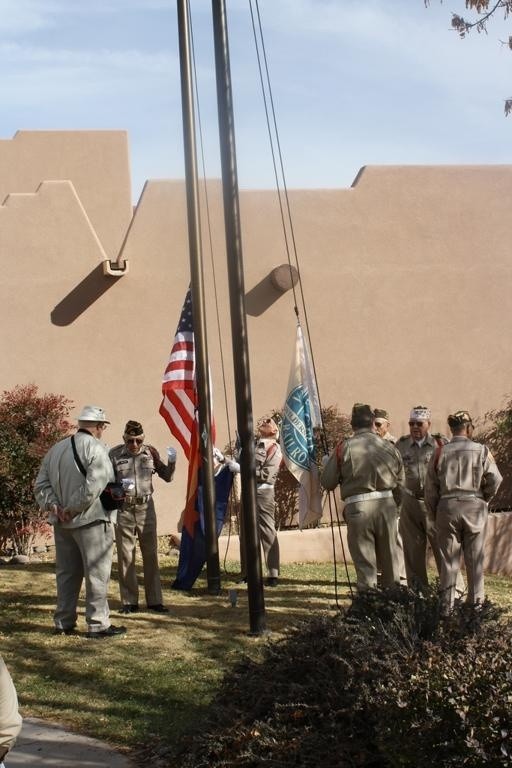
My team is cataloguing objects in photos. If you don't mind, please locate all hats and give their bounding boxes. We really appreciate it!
[271,412,284,430]
[74,406,111,424]
[350,402,376,427]
[373,408,392,424]
[408,407,432,423]
[447,410,473,427]
[124,420,143,436]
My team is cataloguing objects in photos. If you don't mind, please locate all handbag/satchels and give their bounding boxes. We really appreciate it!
[100,482,125,510]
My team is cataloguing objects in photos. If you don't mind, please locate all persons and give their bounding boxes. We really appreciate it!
[425,411,504,617]
[109,421,177,614]
[34,406,126,638]
[212,417,284,587]
[0,653,23,768]
[395,407,469,621]
[372,408,408,586]
[318,403,405,594]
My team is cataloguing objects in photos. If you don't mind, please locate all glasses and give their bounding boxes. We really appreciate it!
[101,424,107,430]
[409,421,429,427]
[374,421,387,427]
[125,438,144,444]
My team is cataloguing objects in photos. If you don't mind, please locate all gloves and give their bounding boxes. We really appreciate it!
[213,448,225,462]
[229,460,240,473]
[165,446,176,464]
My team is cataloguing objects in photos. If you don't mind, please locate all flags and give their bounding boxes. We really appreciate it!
[278,327,325,530]
[157,281,234,592]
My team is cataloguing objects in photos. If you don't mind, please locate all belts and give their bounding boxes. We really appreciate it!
[404,488,426,500]
[344,489,394,506]
[126,494,154,505]
[440,491,485,499]
[256,483,275,489]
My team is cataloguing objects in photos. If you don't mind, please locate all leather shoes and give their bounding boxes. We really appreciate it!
[86,625,127,639]
[117,604,139,614]
[51,628,80,637]
[147,603,171,613]
